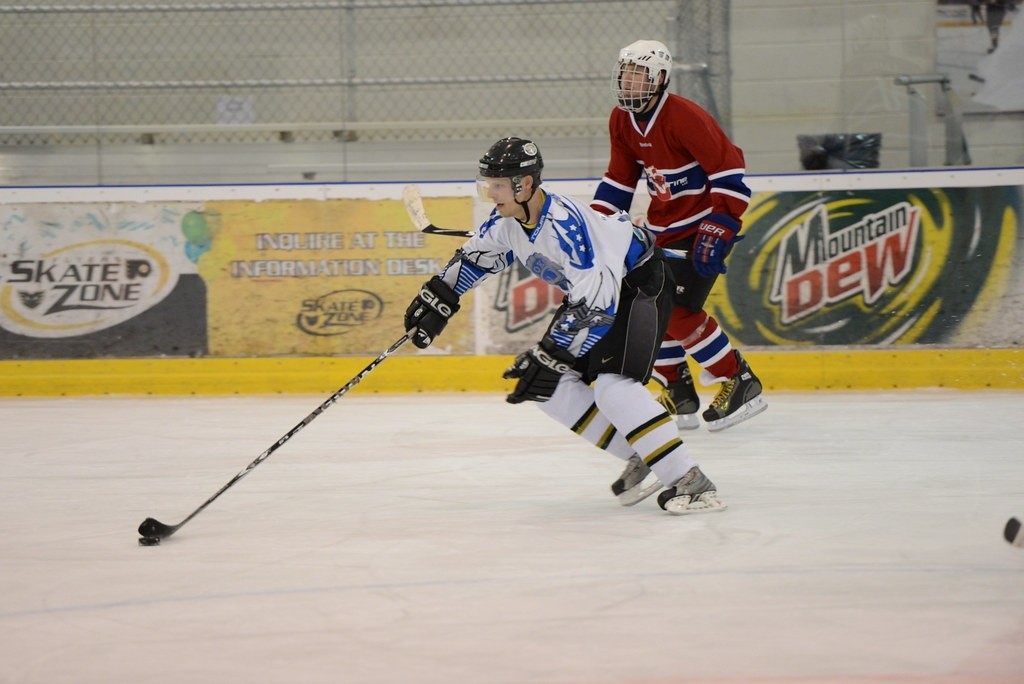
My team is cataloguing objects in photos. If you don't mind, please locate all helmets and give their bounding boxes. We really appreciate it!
[619,40,672,85]
[480,137,544,177]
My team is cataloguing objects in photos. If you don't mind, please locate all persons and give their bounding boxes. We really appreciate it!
[967,0,985,25]
[404,136,728,516]
[982,0,1014,54]
[587,39,768,432]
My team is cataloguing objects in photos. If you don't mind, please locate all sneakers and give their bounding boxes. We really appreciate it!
[703,350,769,432]
[659,468,728,516]
[611,453,664,506]
[657,365,701,429]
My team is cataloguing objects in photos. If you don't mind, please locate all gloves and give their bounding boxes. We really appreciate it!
[504,337,574,403]
[405,275,462,349]
[692,215,746,279]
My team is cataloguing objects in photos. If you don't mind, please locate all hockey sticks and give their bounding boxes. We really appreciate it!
[400,184,694,264]
[135,325,420,545]
[1005,518,1024,548]
